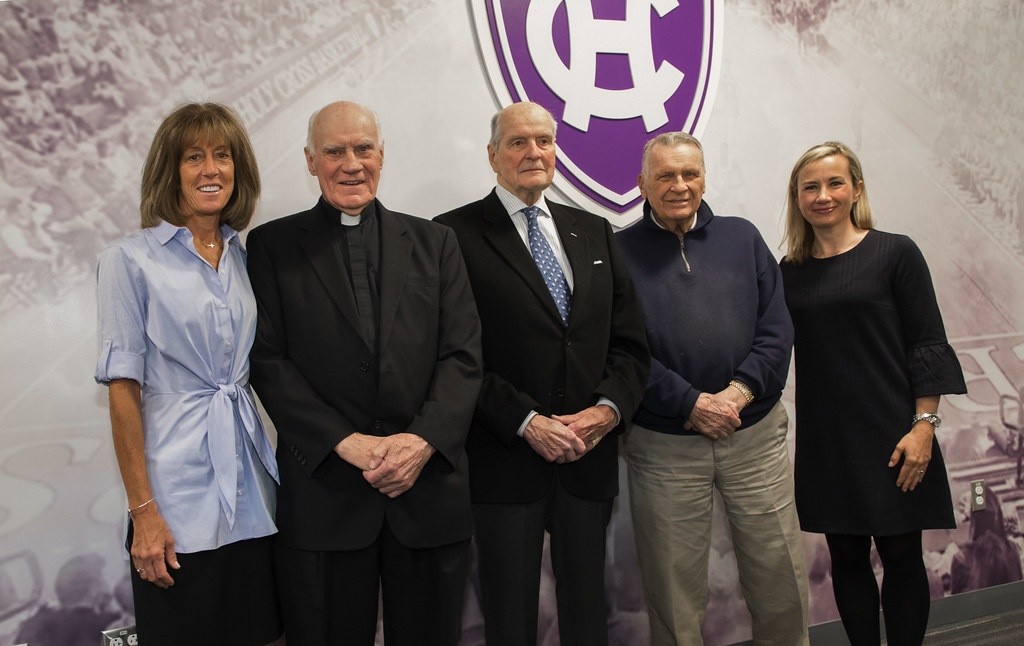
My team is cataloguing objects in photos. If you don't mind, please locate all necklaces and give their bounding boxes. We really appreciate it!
[197,231,222,248]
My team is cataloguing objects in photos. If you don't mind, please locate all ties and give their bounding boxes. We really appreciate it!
[523,206,573,331]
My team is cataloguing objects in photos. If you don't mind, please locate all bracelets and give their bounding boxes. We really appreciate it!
[728,380,754,403]
[128,498,155,513]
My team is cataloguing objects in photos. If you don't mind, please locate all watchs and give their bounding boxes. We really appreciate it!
[912,413,941,427]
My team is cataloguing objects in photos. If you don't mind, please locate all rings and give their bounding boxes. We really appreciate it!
[917,471,923,473]
[137,567,144,572]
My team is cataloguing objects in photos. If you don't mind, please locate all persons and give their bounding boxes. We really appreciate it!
[13,552,107,646]
[428,100,652,646]
[244,100,486,644]
[606,134,813,645]
[92,102,280,646]
[103,575,134,629]
[778,141,968,646]
